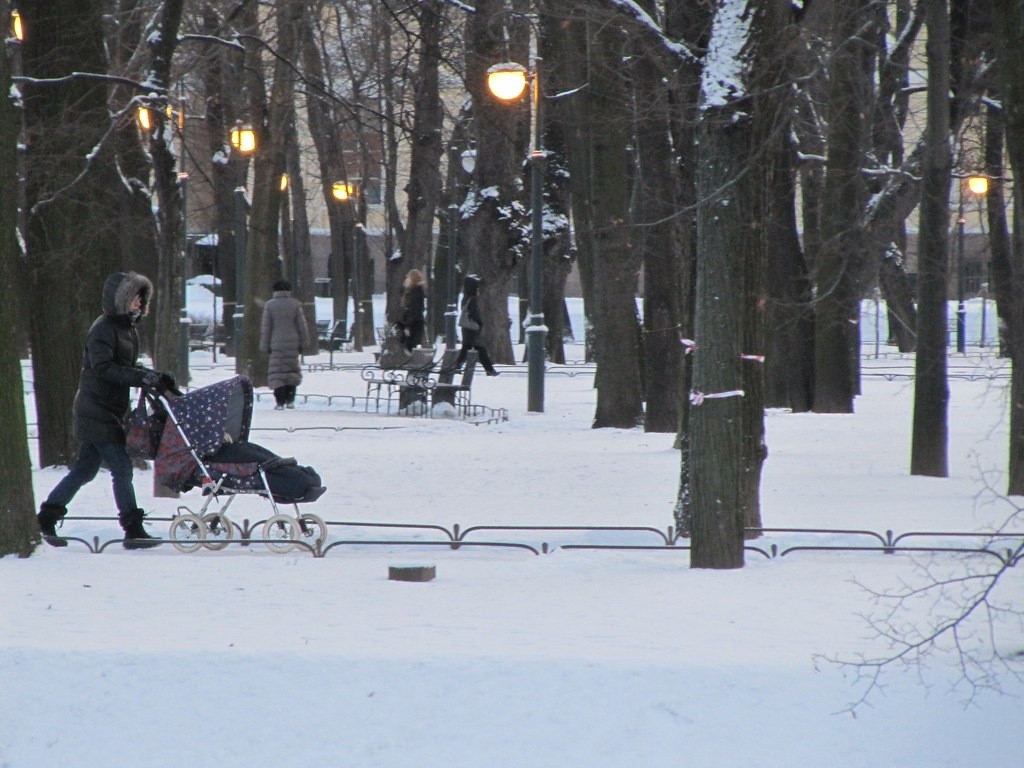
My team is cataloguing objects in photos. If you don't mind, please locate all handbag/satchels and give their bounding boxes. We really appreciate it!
[124,388,168,460]
[458,307,480,330]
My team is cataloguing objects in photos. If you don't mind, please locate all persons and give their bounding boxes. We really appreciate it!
[37,272,176,549]
[204,432,327,501]
[400,268,425,353]
[455,275,502,376]
[258,279,310,410]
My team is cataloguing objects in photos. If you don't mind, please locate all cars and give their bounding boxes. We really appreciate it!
[185,276,228,342]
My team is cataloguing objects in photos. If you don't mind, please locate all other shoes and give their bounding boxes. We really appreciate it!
[274,403,294,410]
[487,369,500,376]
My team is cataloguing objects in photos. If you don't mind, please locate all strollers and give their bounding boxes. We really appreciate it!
[141,375,329,553]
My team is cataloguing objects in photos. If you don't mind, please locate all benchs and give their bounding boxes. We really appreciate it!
[299,319,356,367]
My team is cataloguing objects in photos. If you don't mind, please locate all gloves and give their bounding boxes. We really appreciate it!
[145,370,175,388]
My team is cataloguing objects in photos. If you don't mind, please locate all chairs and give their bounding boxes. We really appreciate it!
[362,349,478,420]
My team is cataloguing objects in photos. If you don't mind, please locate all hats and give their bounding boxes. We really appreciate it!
[272,280,291,290]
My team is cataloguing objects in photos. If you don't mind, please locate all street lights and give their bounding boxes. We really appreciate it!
[957,171,990,354]
[224,115,254,356]
[487,11,549,414]
[442,137,479,348]
[333,170,363,350]
[139,73,191,388]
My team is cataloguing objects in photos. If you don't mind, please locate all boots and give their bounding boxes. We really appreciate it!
[35,502,68,546]
[119,508,163,550]
